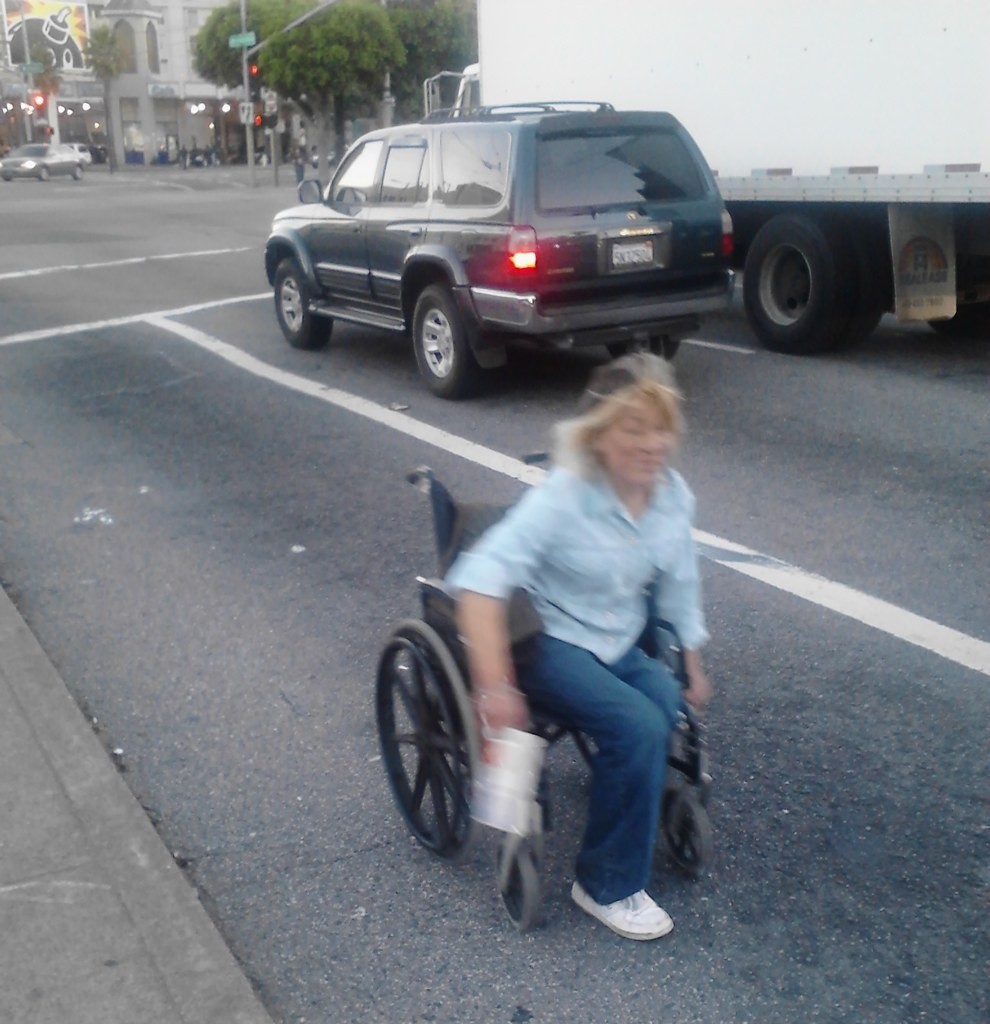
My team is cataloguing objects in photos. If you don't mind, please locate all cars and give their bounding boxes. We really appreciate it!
[0,142,93,183]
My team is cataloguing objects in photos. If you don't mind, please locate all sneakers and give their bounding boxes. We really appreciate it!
[571,880,674,941]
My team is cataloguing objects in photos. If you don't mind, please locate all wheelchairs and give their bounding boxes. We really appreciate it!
[374,451,715,933]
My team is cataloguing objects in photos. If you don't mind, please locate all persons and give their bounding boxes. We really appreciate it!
[289,139,305,182]
[310,145,319,169]
[180,142,221,169]
[329,149,337,168]
[48,127,56,143]
[443,381,712,942]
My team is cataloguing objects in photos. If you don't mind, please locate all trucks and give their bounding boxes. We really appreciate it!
[423,2,990,355]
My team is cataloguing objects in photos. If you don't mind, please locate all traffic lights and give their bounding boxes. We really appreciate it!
[31,92,47,109]
[246,54,261,102]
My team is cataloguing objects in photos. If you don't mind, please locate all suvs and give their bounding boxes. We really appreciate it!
[264,101,738,400]
